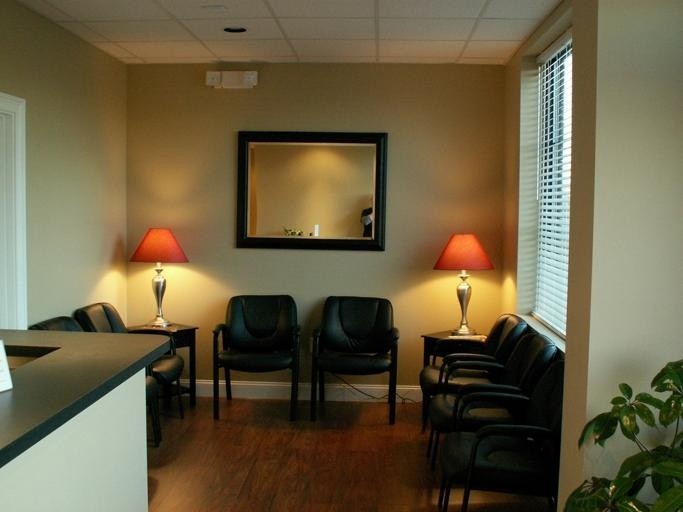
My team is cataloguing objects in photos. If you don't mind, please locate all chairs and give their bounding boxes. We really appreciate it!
[420,314,528,458]
[310,296,398,425]
[212,294,300,422]
[440,360,564,512]
[28,316,160,448]
[429,331,556,508]
[74,303,185,414]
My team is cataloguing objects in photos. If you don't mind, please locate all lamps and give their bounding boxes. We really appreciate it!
[432,233,496,335]
[128,225,189,326]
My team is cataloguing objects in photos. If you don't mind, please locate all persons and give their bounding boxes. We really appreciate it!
[359,195,373,237]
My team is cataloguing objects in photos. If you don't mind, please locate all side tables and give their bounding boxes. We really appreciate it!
[126,322,199,411]
[421,328,489,425]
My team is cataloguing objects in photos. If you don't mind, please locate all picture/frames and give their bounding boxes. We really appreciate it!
[236,130,389,252]
